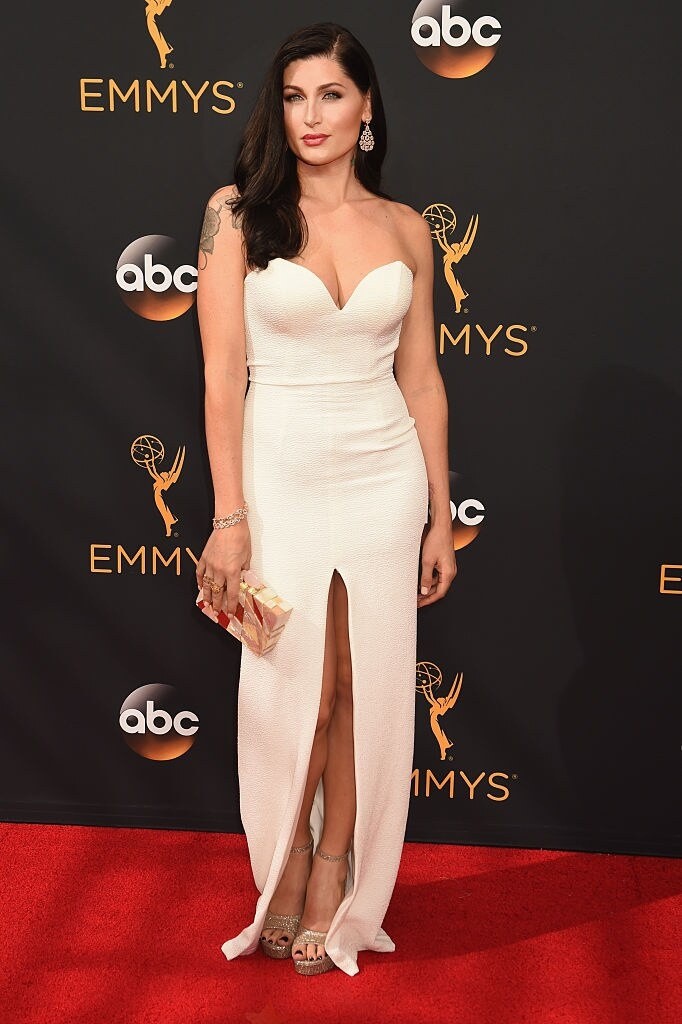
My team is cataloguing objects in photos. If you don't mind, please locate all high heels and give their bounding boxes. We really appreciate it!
[262,837,314,959]
[291,838,353,975]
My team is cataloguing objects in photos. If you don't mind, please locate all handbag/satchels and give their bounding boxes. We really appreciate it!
[195,568,292,657]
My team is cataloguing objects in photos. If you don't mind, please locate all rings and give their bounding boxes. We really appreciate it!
[203,574,214,580]
[211,582,222,593]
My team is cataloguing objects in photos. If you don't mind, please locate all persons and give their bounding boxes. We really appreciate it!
[193,22,455,978]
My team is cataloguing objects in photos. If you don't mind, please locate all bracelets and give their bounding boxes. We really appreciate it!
[212,501,248,528]
